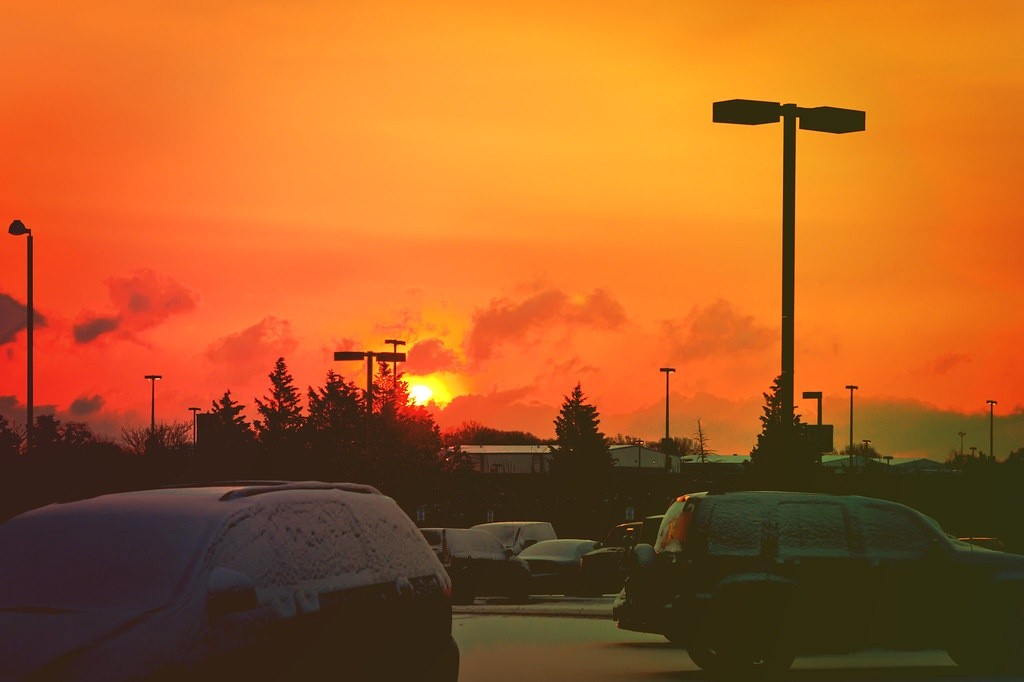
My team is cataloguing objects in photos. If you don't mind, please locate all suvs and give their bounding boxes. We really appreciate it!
[0,481,460,682]
[612,490,1024,682]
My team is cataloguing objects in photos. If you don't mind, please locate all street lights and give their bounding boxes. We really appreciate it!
[384,338,407,386]
[845,385,858,453]
[145,373,162,432]
[986,399,997,460]
[862,440,871,465]
[188,406,198,442]
[802,391,823,489]
[634,440,644,475]
[7,221,34,457]
[958,431,966,455]
[658,367,676,436]
[334,352,406,486]
[713,98,865,415]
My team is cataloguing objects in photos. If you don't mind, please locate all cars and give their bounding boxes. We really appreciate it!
[415,516,668,606]
